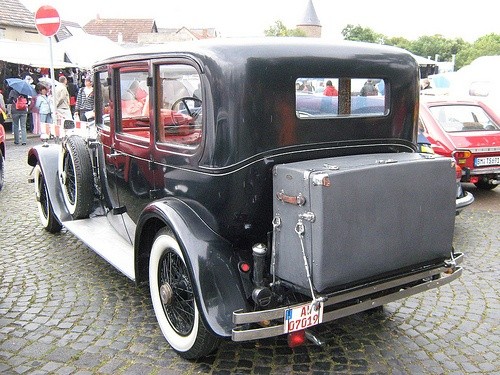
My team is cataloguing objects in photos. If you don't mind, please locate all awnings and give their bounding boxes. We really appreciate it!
[0,39,79,69]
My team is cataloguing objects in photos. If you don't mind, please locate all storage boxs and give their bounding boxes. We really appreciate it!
[273,151,457,293]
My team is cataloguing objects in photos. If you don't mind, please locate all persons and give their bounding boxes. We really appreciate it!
[303,79,433,96]
[0,67,201,146]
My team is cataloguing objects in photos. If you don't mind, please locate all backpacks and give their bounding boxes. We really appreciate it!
[13,95,28,111]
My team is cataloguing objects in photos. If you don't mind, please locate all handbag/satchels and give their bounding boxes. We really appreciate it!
[2,111,7,120]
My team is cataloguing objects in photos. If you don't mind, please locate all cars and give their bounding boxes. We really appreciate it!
[27,37,465,361]
[294,54,500,192]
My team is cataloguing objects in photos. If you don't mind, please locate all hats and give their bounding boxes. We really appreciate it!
[85,75,93,82]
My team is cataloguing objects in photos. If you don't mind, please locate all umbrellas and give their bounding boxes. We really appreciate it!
[38,77,59,86]
[5,78,38,97]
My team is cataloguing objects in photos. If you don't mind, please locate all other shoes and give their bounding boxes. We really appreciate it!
[14,142,19,144]
[22,143,26,145]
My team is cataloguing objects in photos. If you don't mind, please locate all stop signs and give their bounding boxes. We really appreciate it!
[35,4,61,37]
